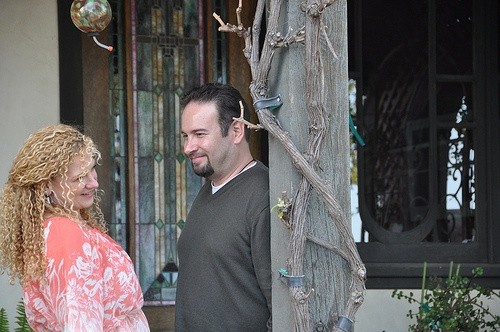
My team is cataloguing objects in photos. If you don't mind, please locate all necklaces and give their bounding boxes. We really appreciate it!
[239,159,254,173]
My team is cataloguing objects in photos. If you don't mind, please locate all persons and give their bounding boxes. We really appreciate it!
[0,124,151,332]
[175,83,273,332]
